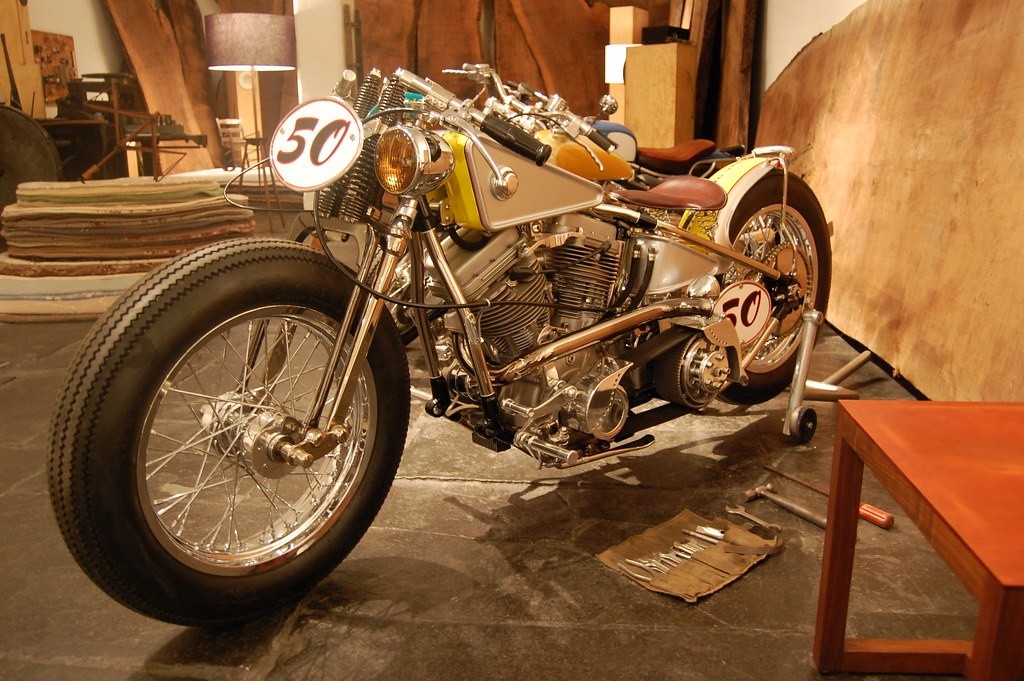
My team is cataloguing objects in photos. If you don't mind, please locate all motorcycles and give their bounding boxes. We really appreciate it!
[45,63,833,631]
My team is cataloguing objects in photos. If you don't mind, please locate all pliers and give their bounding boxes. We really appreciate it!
[619,556,657,584]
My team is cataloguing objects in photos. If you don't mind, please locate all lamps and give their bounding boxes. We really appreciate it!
[203,14,297,233]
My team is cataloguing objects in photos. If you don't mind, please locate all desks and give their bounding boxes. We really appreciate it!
[812,399,1024,681]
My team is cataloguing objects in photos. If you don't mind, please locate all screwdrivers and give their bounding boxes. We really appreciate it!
[762,462,895,531]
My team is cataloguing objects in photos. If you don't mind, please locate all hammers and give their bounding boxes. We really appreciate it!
[743,482,827,538]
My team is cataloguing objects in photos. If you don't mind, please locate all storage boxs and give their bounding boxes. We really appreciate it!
[625,44,697,146]
[641,25,690,44]
[609,83,625,126]
[610,7,648,44]
[605,45,642,84]
